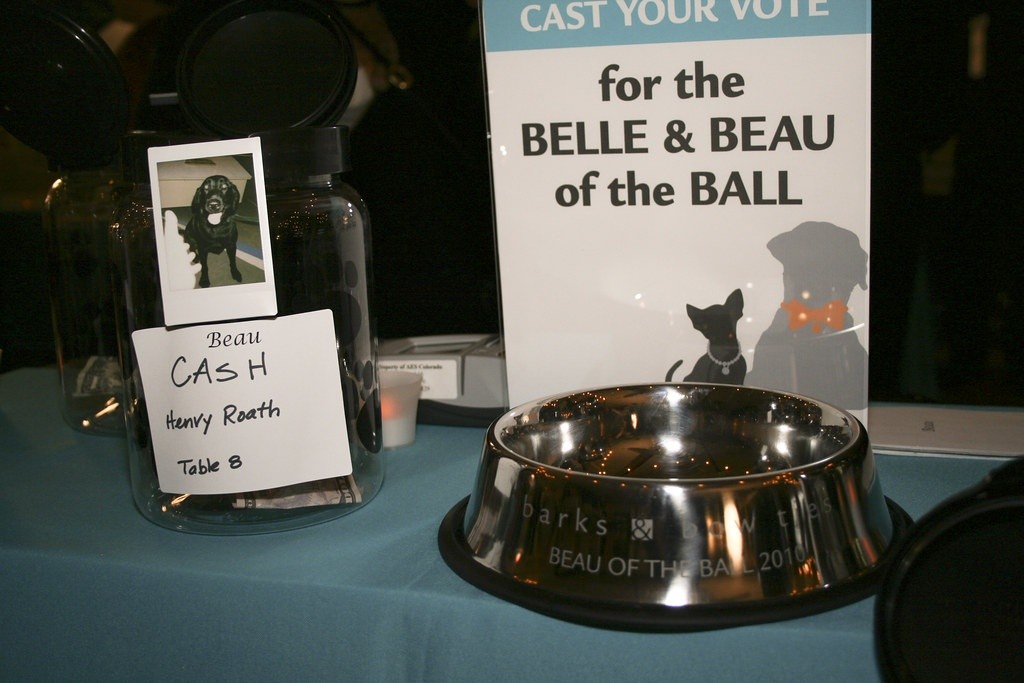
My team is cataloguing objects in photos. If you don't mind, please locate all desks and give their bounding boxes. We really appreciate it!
[0,351,1024,683]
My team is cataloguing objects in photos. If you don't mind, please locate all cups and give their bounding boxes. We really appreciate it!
[378,369,426,449]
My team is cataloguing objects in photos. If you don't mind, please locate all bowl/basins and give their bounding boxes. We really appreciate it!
[439,382,914,631]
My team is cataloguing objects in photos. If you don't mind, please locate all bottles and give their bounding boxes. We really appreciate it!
[42,161,130,438]
[111,128,386,535]
